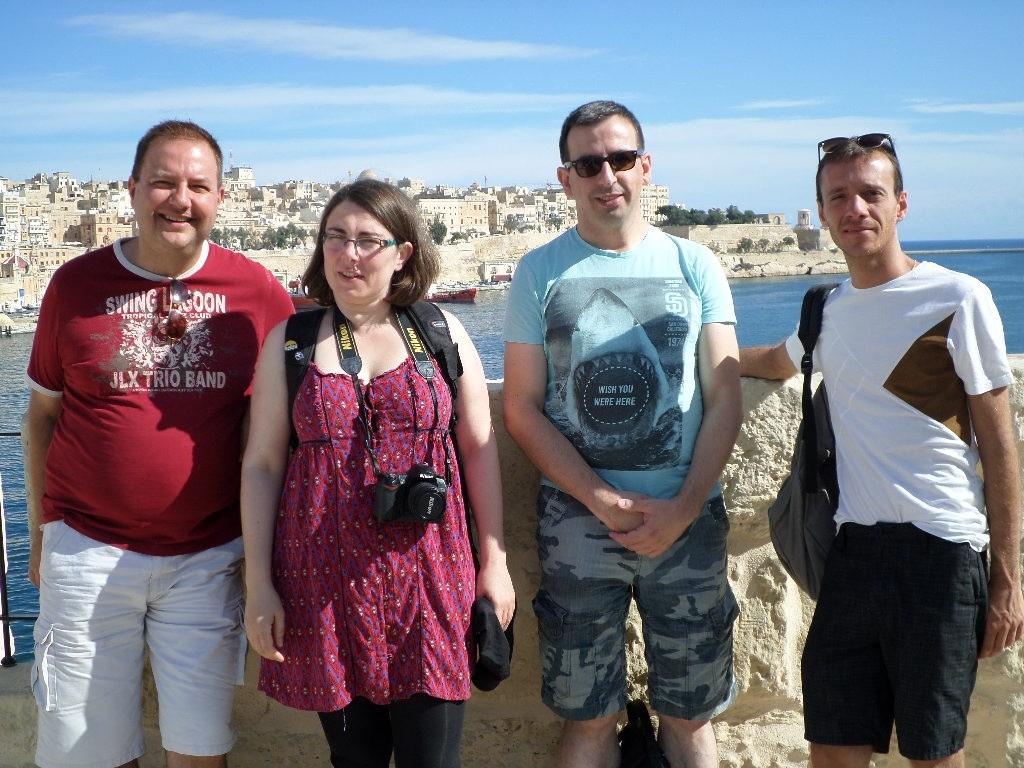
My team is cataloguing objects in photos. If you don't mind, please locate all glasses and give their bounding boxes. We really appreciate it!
[165,277,189,340]
[818,132,897,164]
[563,150,643,178]
[322,232,399,256]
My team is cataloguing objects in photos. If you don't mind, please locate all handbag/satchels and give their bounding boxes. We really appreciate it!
[767,281,840,600]
[615,700,670,768]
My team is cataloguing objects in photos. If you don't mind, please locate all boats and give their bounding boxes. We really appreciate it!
[422,288,476,304]
[288,283,316,309]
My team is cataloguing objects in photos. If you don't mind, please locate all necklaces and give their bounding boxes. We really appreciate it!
[912,260,916,269]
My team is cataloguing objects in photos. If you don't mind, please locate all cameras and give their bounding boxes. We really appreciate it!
[374,462,449,523]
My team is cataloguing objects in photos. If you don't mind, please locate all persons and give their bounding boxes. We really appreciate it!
[26,121,295,768]
[502,102,744,768]
[240,181,515,768]
[738,133,1024,768]
